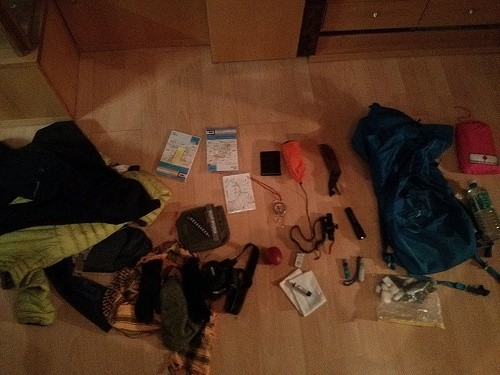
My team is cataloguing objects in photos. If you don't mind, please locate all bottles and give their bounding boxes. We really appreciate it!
[466,179,500,241]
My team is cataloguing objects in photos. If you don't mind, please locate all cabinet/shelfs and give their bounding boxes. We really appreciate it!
[298,0,500,56]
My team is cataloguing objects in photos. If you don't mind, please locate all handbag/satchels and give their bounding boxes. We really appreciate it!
[200,241,259,315]
[349,100,479,277]
